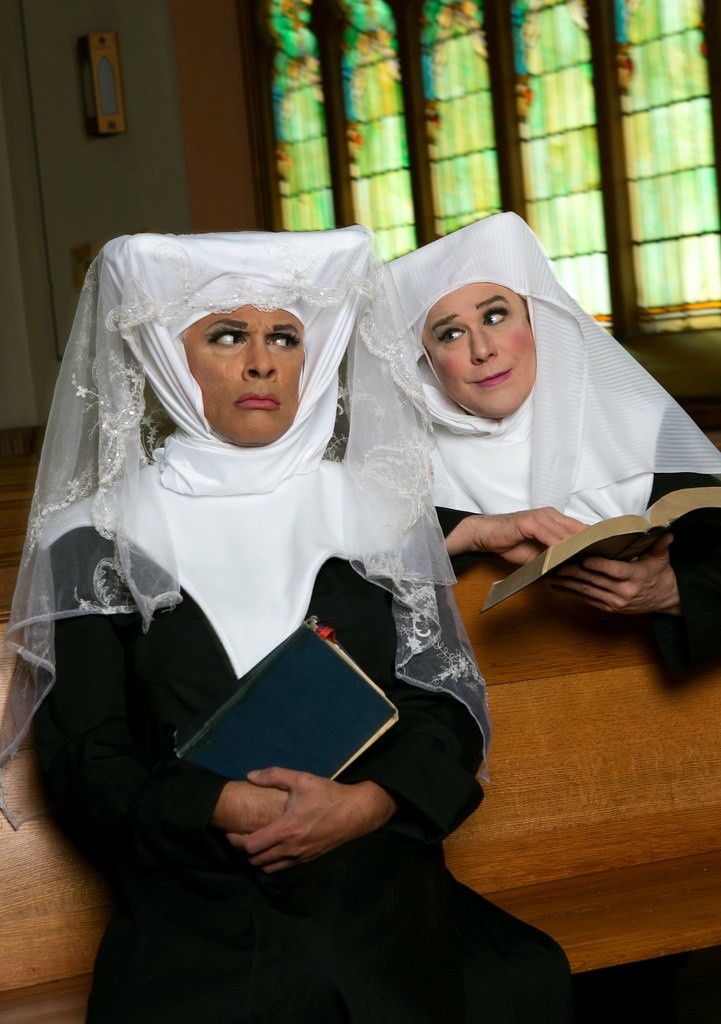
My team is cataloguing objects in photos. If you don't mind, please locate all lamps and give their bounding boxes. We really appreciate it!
[76,30,126,137]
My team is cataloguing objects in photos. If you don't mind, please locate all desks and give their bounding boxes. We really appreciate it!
[0,462,721,1024]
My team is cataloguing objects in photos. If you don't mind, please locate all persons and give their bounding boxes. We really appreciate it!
[381,212,720,692]
[1,224,577,1024]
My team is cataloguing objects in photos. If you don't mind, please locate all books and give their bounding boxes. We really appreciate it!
[176,613,399,790]
[480,488,721,615]
